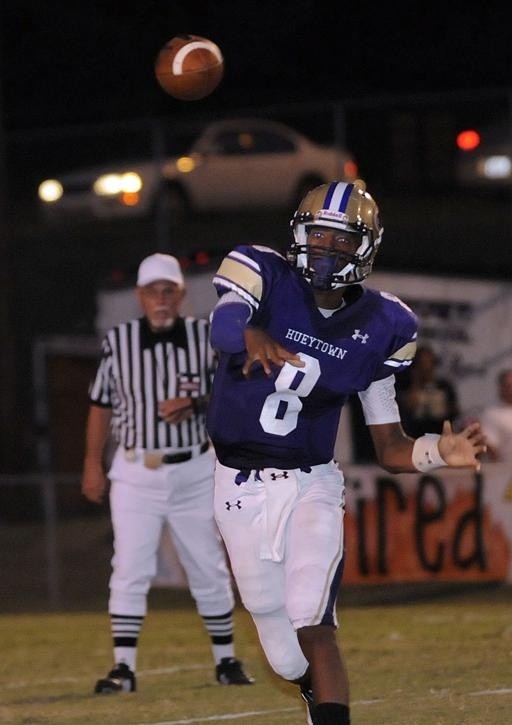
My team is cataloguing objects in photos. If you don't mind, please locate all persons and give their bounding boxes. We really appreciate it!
[465,368,511,461]
[203,180,485,725]
[397,344,461,438]
[84,251,256,695]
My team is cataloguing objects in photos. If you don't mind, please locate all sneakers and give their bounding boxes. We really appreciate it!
[301,677,317,724]
[214,655,254,685]
[94,661,137,695]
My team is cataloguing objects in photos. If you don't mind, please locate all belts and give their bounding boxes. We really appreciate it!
[144,441,212,468]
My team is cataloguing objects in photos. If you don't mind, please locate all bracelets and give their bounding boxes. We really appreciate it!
[188,396,206,415]
[411,431,445,473]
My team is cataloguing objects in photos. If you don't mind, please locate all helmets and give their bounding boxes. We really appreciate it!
[289,179,384,291]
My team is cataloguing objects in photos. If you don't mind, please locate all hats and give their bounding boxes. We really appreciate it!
[136,251,184,288]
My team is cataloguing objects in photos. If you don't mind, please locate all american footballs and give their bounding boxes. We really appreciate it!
[156,36,224,101]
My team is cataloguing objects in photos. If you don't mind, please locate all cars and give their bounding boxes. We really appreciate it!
[38,121,355,221]
